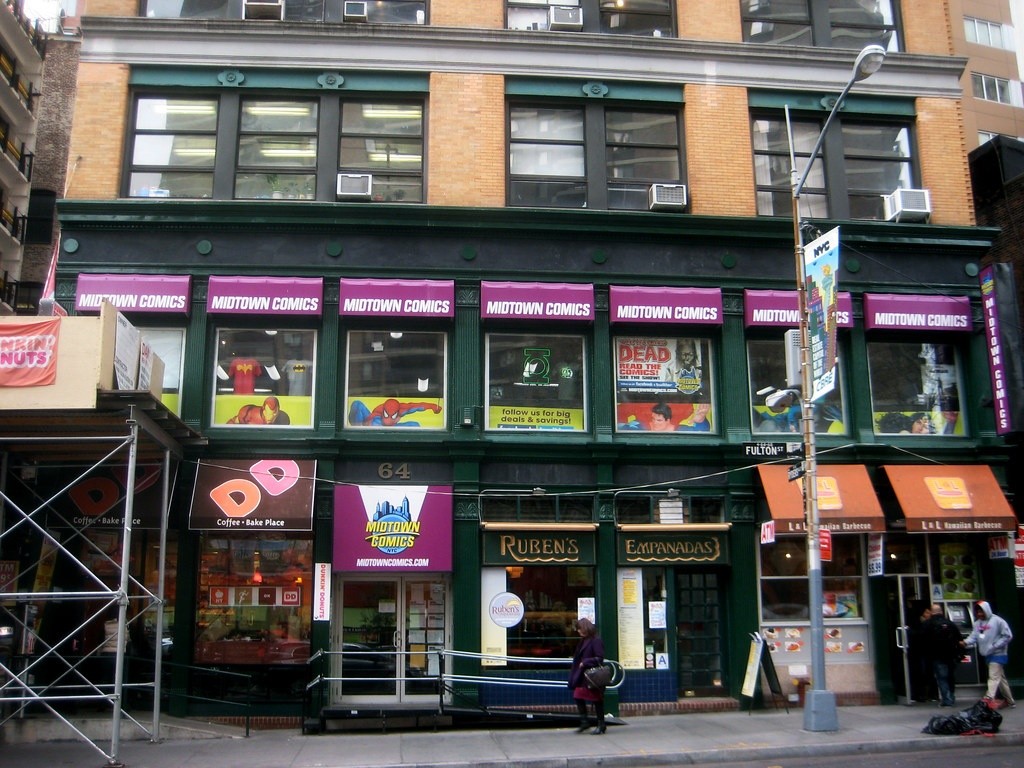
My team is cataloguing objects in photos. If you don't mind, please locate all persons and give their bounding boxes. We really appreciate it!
[281,353,313,396]
[874,411,959,435]
[568,618,606,735]
[229,348,262,395]
[959,601,1016,708]
[919,605,963,706]
[665,338,702,383]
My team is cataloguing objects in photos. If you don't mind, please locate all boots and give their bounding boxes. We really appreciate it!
[576,716,591,734]
[591,719,606,735]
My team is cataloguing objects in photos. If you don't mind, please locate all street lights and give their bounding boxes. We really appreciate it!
[765,44,886,733]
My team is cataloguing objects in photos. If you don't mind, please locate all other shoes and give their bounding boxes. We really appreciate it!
[938,702,953,709]
[1007,703,1016,707]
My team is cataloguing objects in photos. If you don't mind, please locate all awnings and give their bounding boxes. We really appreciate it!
[878,465,1020,534]
[757,464,886,537]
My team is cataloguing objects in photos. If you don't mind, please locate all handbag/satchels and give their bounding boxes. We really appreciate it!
[945,634,968,658]
[584,657,612,690]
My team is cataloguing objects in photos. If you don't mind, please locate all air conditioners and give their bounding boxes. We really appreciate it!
[648,184,687,212]
[880,188,933,224]
[344,1,368,22]
[547,6,583,31]
[337,173,372,202]
